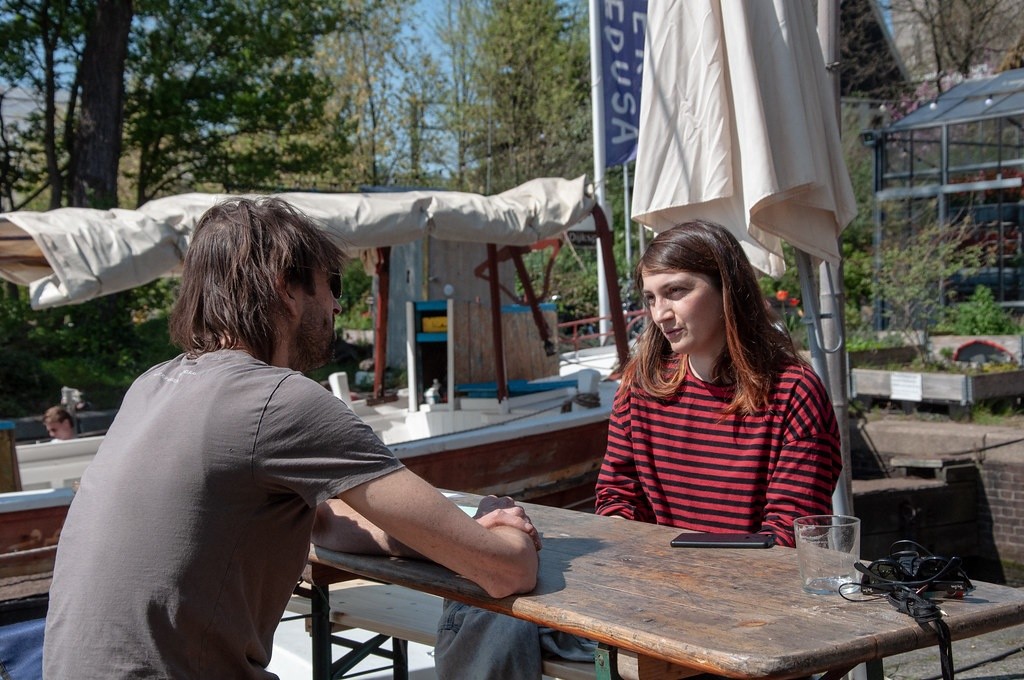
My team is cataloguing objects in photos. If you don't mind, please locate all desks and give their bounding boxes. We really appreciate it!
[304,486,1024,680]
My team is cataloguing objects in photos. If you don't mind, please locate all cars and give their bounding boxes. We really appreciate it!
[945,240,1024,300]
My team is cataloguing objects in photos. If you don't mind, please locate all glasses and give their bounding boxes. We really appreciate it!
[287,266,343,299]
[854,557,973,593]
[47,423,61,435]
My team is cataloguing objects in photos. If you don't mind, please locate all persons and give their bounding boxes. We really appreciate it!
[44,406,76,442]
[44,198,542,680]
[595,219,841,547]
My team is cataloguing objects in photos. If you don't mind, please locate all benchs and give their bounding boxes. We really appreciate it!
[283,580,637,680]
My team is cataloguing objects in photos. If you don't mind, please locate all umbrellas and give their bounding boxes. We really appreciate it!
[631,1,859,559]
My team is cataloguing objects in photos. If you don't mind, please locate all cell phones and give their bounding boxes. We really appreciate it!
[670,533,776,549]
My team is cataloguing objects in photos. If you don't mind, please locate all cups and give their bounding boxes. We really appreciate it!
[794,514,862,597]
